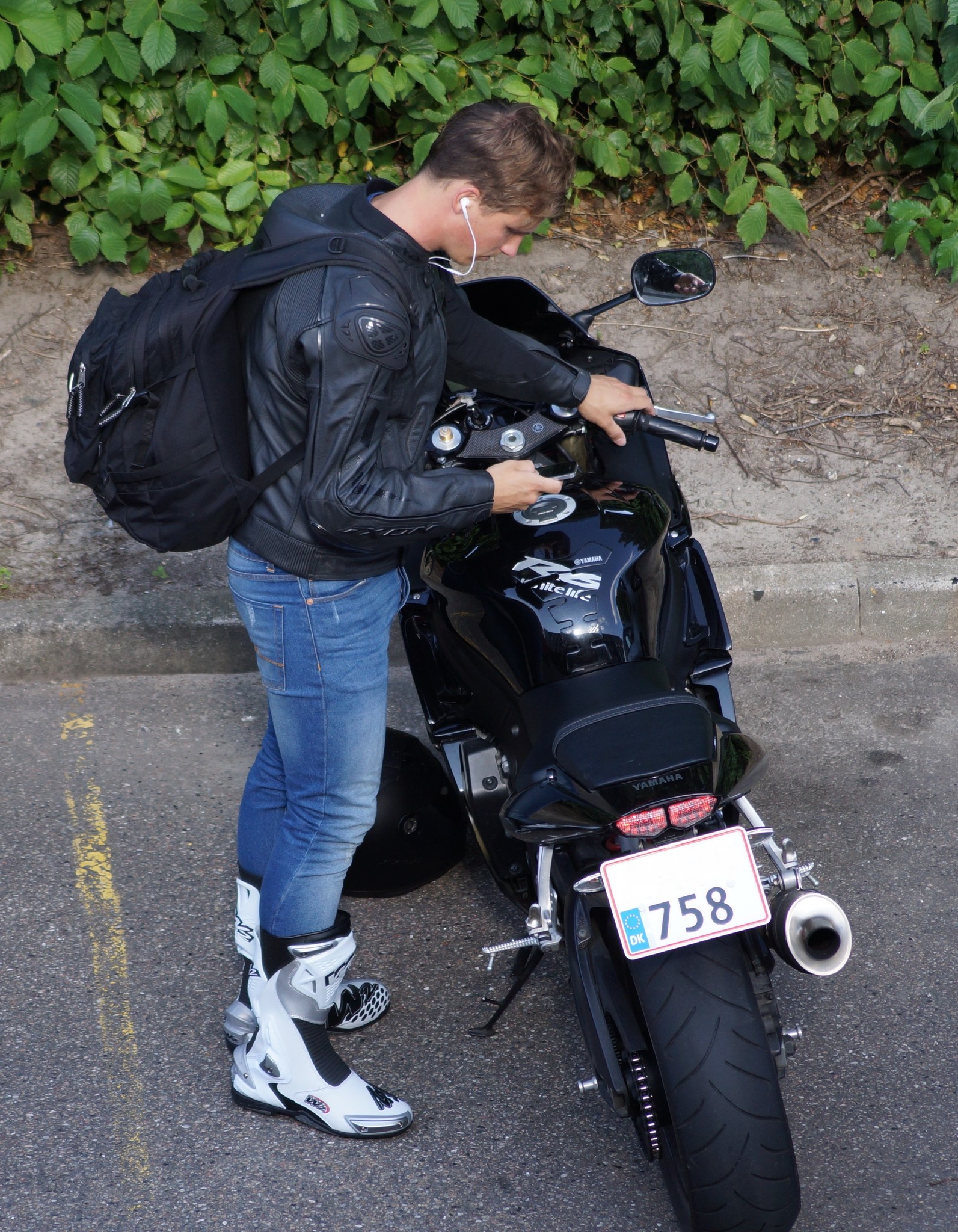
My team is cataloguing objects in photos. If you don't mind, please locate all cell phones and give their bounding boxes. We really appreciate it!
[679,283,713,290]
[535,462,581,479]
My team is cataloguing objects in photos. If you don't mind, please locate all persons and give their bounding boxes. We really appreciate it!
[634,253,711,297]
[212,96,659,1153]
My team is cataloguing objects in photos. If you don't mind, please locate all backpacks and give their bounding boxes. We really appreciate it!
[62,231,418,554]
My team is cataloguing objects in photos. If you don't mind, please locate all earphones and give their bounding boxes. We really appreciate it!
[460,197,469,220]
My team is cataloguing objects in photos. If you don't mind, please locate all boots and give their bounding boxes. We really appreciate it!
[230,909,414,1138]
[223,875,391,1055]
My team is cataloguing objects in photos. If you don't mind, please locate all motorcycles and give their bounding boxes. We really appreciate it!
[394,246,856,1232]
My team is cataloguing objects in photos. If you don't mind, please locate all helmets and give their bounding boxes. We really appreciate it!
[342,727,467,899]
